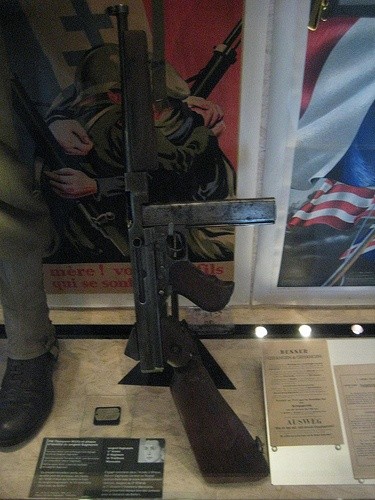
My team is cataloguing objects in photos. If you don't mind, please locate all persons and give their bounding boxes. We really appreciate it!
[1,78,61,449]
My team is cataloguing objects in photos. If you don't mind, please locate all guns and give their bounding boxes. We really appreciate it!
[106,2,278,486]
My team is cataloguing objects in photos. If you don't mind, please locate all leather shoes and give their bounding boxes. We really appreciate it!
[0,341,58,447]
